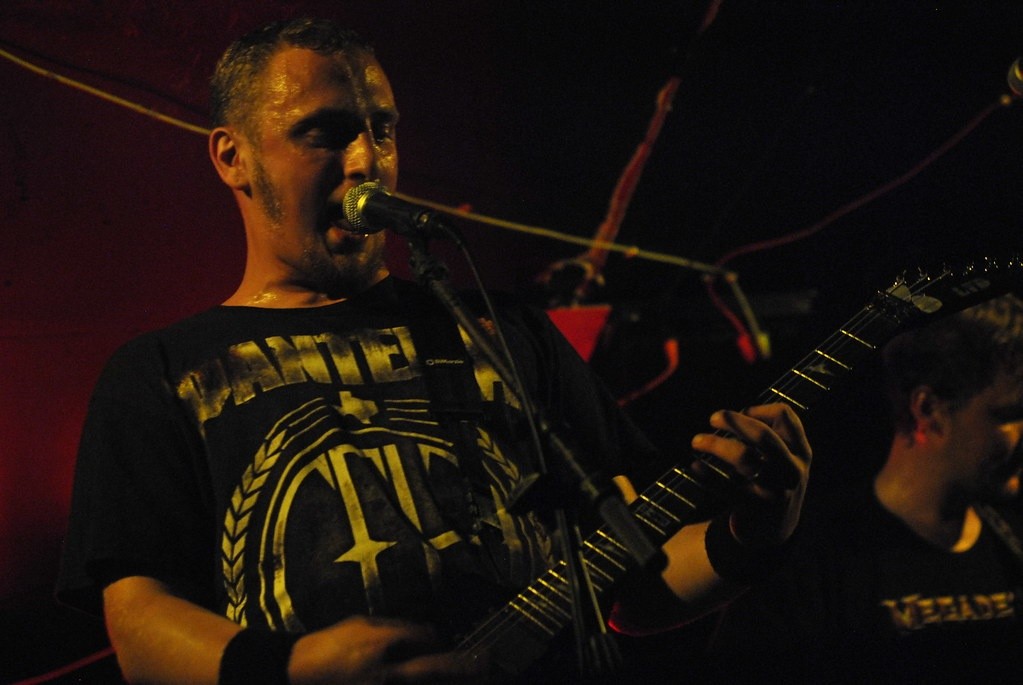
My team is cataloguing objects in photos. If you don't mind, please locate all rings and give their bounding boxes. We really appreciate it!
[747,461,766,482]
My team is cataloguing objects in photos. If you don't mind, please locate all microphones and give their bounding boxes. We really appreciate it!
[339,181,440,235]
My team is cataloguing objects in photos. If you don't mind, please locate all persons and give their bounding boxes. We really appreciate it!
[791,292,1023,684]
[65,16,817,685]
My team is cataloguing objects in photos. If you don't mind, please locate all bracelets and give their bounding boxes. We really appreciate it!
[216,628,299,685]
[706,510,751,580]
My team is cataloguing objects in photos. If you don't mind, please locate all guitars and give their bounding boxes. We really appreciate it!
[453,251,1023,684]
